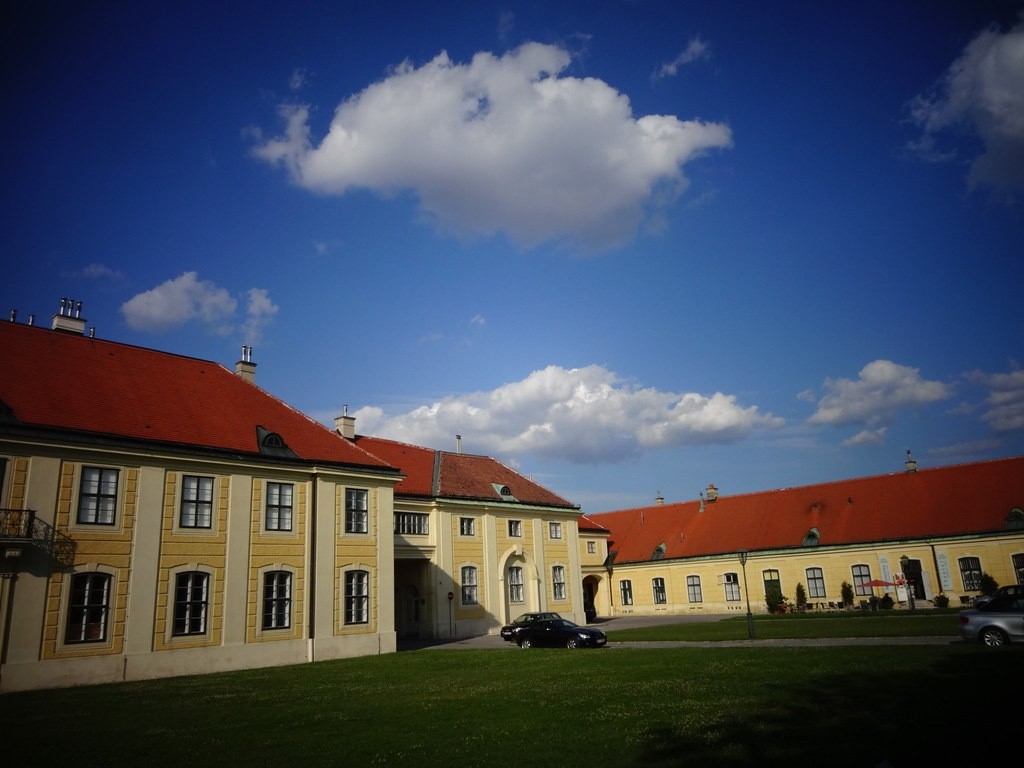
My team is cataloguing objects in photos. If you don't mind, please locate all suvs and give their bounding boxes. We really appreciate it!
[500,611,562,641]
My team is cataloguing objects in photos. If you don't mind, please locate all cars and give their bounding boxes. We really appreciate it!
[973,584,1024,610]
[515,618,608,650]
[959,592,1024,648]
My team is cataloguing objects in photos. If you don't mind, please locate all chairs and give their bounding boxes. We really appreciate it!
[860,600,872,612]
[959,596,974,607]
[806,601,846,614]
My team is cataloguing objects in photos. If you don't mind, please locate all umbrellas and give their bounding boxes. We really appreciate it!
[858,579,898,598]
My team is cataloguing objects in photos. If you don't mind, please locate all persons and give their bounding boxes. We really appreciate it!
[778,597,791,614]
[885,593,893,610]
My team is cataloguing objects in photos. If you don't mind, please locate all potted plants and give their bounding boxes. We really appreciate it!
[934,595,949,608]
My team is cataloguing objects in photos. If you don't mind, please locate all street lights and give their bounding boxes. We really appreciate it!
[736,546,755,638]
[900,554,915,609]
